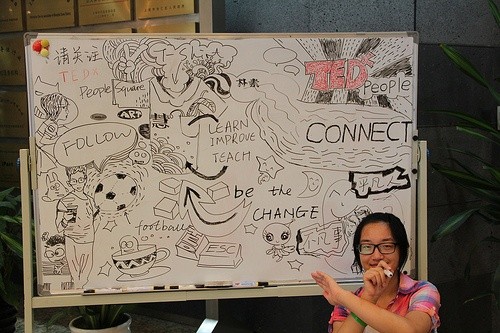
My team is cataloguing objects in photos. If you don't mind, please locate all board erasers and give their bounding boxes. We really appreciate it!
[204,280,234,288]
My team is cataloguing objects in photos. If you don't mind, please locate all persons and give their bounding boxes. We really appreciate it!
[311,212,441,332]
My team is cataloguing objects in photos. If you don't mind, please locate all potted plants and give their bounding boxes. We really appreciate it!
[0,186,37,333]
[44,302,138,333]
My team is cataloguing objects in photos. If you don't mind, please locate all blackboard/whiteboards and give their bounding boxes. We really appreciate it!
[20,29,420,299]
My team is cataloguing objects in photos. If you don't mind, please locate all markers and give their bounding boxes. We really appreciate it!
[83,287,124,294]
[382,268,393,278]
[239,281,270,287]
[169,284,205,290]
[126,284,166,292]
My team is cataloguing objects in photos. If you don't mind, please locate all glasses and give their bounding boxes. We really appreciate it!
[356,242,397,254]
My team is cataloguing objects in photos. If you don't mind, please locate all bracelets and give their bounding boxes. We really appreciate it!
[351,311,368,328]
